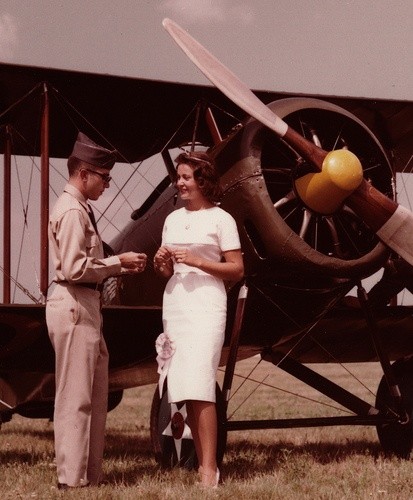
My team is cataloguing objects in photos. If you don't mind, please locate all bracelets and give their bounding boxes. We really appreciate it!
[154,255,166,271]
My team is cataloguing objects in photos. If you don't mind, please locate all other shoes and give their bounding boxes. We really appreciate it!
[194,467,220,489]
[57,482,90,490]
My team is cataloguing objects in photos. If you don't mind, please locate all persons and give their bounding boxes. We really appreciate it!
[154,152,244,489]
[45,130,147,491]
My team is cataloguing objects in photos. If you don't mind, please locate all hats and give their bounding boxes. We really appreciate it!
[71,130,119,170]
[184,152,214,168]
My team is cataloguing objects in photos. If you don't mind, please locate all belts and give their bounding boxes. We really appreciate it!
[80,282,105,292]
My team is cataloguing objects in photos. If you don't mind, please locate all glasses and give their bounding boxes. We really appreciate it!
[79,168,112,183]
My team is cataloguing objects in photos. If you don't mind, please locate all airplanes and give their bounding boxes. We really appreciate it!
[0,0,413,460]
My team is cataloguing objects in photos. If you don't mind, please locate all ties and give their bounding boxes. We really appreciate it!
[87,204,98,234]
